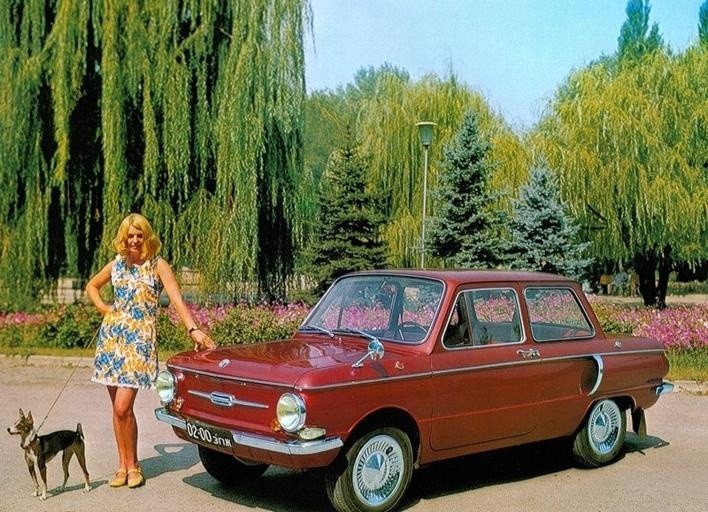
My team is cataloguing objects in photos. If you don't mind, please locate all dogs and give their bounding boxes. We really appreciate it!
[7,406,91,501]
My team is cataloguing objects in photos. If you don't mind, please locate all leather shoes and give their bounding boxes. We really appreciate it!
[127,466,144,488]
[110,468,127,486]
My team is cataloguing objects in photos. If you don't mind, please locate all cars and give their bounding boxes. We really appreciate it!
[153,268,675,512]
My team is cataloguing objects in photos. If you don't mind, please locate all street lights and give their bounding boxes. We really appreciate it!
[413,121,439,269]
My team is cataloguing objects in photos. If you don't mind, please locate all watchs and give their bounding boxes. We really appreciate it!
[189,326,200,334]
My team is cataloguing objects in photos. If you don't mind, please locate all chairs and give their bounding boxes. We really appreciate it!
[383,320,589,346]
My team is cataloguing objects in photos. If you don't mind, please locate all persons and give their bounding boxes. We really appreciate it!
[86,213,217,488]
[610,266,627,296]
